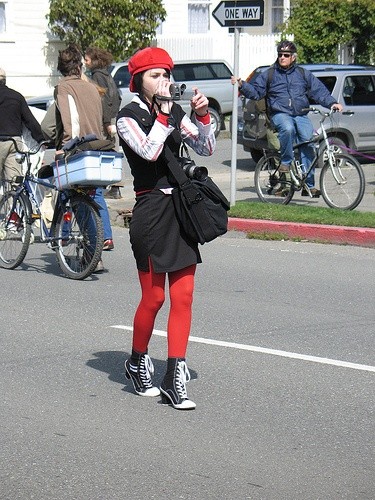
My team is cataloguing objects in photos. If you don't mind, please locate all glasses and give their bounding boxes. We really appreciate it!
[278,53,293,58]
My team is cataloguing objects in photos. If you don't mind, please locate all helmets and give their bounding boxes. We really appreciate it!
[277,39,297,54]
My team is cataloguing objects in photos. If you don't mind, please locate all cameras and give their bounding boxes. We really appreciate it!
[167,158,208,184]
[170,83,186,96]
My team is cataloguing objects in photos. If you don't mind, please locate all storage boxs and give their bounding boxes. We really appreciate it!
[51,150,124,190]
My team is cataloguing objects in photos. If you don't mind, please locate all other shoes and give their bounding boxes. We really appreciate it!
[52,239,68,247]
[301,187,322,196]
[101,239,115,250]
[279,164,290,173]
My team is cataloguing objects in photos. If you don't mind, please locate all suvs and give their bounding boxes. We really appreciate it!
[24,60,234,139]
[231,64,375,168]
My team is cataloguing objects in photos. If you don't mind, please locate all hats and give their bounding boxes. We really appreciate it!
[128,47,174,93]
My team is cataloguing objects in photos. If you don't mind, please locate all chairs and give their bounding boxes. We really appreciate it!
[350,85,370,105]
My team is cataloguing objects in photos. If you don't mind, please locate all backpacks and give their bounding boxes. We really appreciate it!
[241,66,308,142]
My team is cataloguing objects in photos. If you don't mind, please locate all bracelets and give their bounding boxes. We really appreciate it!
[195,110,208,117]
[160,110,170,117]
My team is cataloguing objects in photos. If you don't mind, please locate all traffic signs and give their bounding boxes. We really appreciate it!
[212,0,265,28]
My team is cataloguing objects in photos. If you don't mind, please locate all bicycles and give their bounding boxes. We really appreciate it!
[0,137,105,280]
[254,105,365,210]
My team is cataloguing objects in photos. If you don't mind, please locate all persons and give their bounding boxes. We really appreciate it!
[53,48,114,250]
[41,74,105,249]
[231,41,343,196]
[84,48,122,199]
[115,47,217,410]
[0,67,48,244]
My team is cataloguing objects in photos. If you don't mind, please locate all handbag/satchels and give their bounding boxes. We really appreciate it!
[170,175,231,246]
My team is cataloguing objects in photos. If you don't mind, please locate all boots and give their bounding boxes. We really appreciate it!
[158,356,197,410]
[124,347,161,397]
